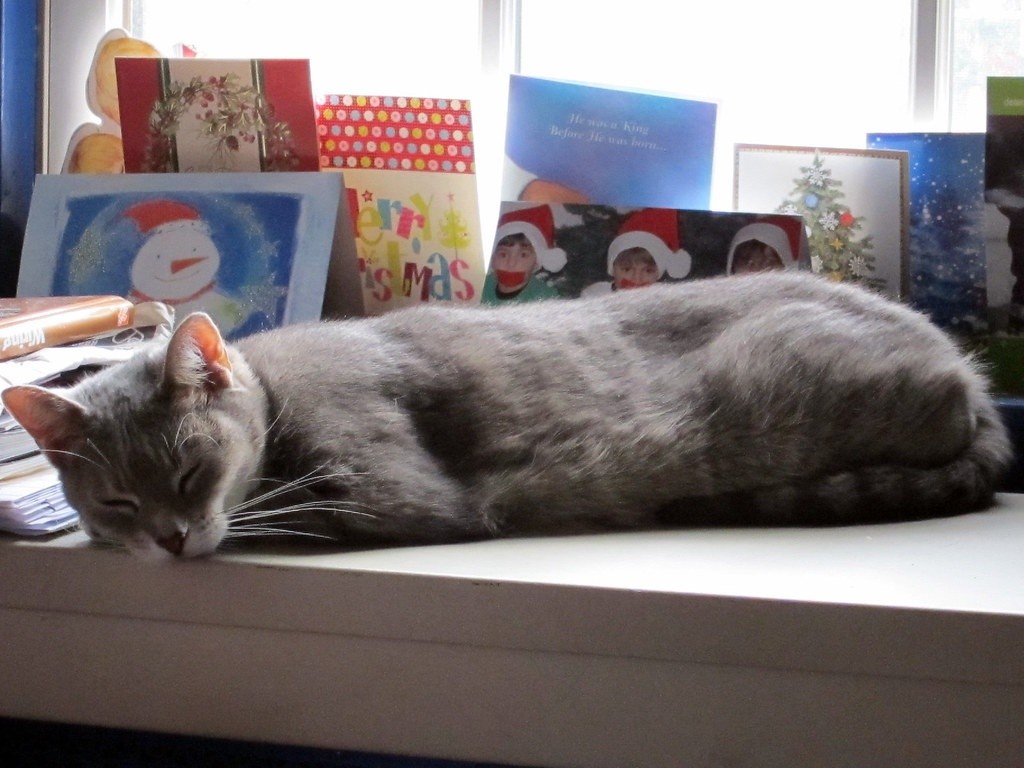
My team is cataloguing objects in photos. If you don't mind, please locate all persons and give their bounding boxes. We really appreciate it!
[581,208,693,304]
[476,205,567,307]
[727,213,804,283]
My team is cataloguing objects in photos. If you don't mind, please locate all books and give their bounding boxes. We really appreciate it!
[1,294,173,540]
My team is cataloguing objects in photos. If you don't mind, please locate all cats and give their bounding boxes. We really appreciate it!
[1,262,1012,560]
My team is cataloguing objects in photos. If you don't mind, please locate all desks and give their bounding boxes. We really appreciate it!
[0,488,1024,768]
